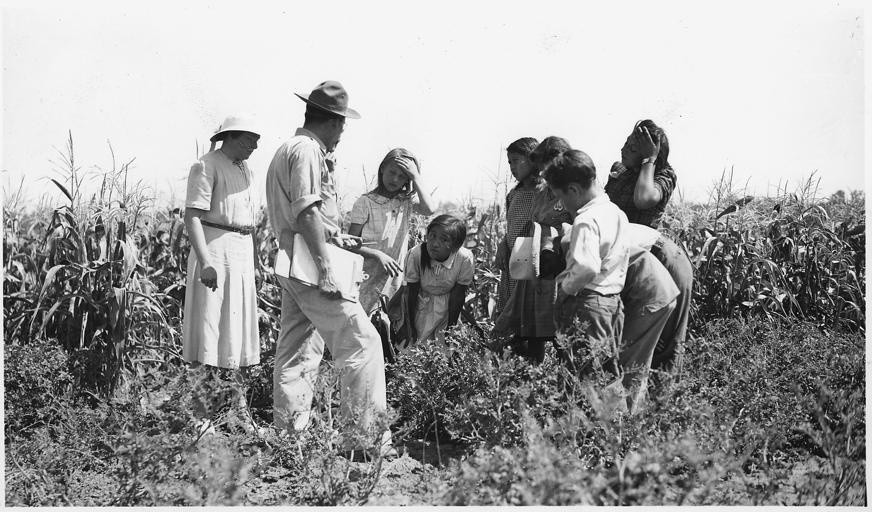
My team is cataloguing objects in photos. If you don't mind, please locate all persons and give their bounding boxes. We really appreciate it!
[264,78,417,465]
[485,122,695,415]
[175,115,270,435]
[398,209,476,348]
[346,144,435,365]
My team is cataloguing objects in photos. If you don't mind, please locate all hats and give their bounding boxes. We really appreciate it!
[293,81,362,121]
[508,221,542,296]
[210,116,262,142]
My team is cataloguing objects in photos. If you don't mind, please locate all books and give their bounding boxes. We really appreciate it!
[290,233,359,299]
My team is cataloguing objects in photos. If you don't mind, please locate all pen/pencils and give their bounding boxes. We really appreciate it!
[361,241,378,245]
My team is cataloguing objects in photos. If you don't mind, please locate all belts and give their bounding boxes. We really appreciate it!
[650,233,668,255]
[200,220,254,236]
